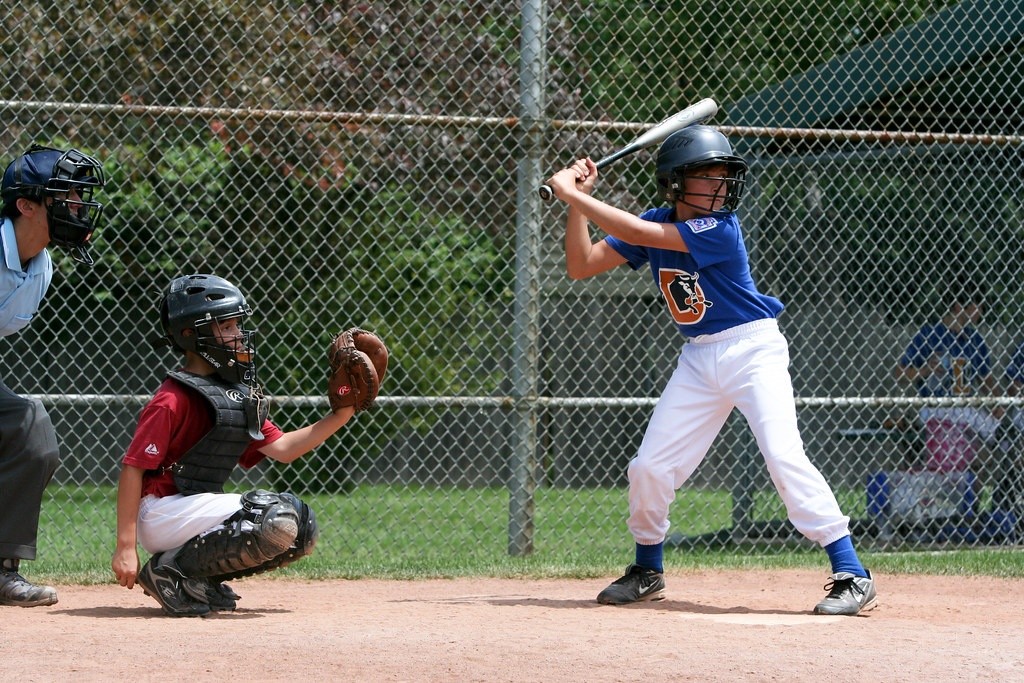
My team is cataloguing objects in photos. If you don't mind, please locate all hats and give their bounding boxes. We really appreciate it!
[2,146,98,203]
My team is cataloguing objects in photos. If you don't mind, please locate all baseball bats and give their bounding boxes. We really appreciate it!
[538,96,720,203]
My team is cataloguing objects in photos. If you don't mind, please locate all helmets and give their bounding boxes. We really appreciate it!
[159,275,259,386]
[656,124,747,201]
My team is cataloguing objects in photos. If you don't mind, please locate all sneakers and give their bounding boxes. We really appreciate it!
[193,576,236,611]
[136,552,210,618]
[0,559,57,607]
[813,568,879,615]
[597,563,665,606]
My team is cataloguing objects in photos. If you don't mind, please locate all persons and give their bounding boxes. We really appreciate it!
[545,123,879,615]
[896,277,1024,505]
[0,144,106,607]
[112,275,390,615]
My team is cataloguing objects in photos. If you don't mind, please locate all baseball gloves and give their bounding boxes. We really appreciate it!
[324,325,390,417]
